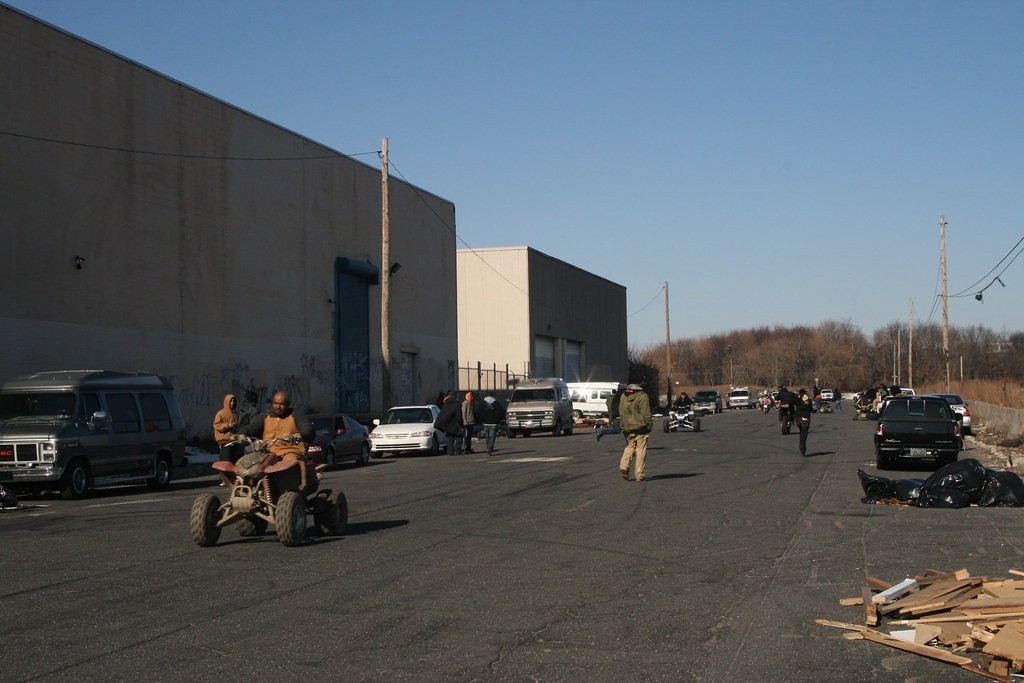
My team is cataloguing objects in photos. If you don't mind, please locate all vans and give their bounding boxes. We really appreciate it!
[691,389,723,414]
[504,377,574,438]
[566,380,619,423]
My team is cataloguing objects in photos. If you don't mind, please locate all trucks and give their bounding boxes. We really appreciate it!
[725,387,760,411]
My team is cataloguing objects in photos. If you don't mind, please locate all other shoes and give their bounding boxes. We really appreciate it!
[596,430,601,442]
[636,478,644,482]
[621,467,629,480]
[450,449,474,455]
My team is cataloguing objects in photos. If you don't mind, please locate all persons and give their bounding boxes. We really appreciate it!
[605,388,616,426]
[434,390,464,455]
[890,386,907,396]
[437,392,445,409]
[832,388,841,412]
[813,386,821,399]
[761,394,771,404]
[482,391,505,455]
[865,382,888,413]
[231,390,314,461]
[213,394,240,486]
[462,392,476,454]
[673,392,693,413]
[619,375,653,481]
[596,378,628,444]
[793,388,817,455]
[775,386,795,413]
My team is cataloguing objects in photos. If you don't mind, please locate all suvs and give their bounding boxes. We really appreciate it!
[0,368,187,501]
[874,395,965,471]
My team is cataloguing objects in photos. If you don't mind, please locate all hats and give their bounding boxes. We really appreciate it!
[466,392,472,400]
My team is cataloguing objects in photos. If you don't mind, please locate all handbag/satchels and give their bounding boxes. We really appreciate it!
[796,416,809,428]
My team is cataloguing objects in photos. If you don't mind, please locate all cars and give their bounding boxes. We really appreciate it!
[295,411,372,471]
[852,387,971,435]
[820,388,835,402]
[369,403,448,458]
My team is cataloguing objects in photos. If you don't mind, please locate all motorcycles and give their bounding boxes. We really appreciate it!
[663,403,701,433]
[190,435,349,547]
[758,387,834,436]
